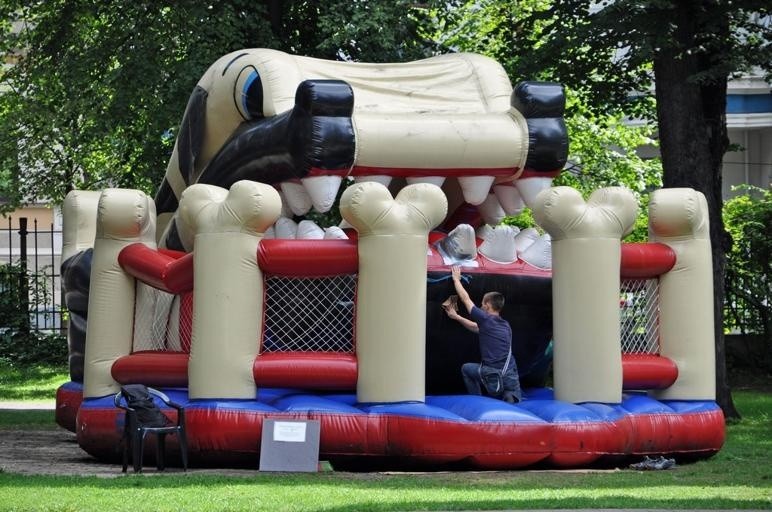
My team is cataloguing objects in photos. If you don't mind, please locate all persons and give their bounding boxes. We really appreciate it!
[444,263,525,404]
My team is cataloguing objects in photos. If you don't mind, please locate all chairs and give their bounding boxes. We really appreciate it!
[115,386,186,474]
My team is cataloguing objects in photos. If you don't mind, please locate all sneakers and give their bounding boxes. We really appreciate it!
[630,455,675,470]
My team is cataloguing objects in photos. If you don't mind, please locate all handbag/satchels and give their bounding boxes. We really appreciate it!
[481,371,504,397]
